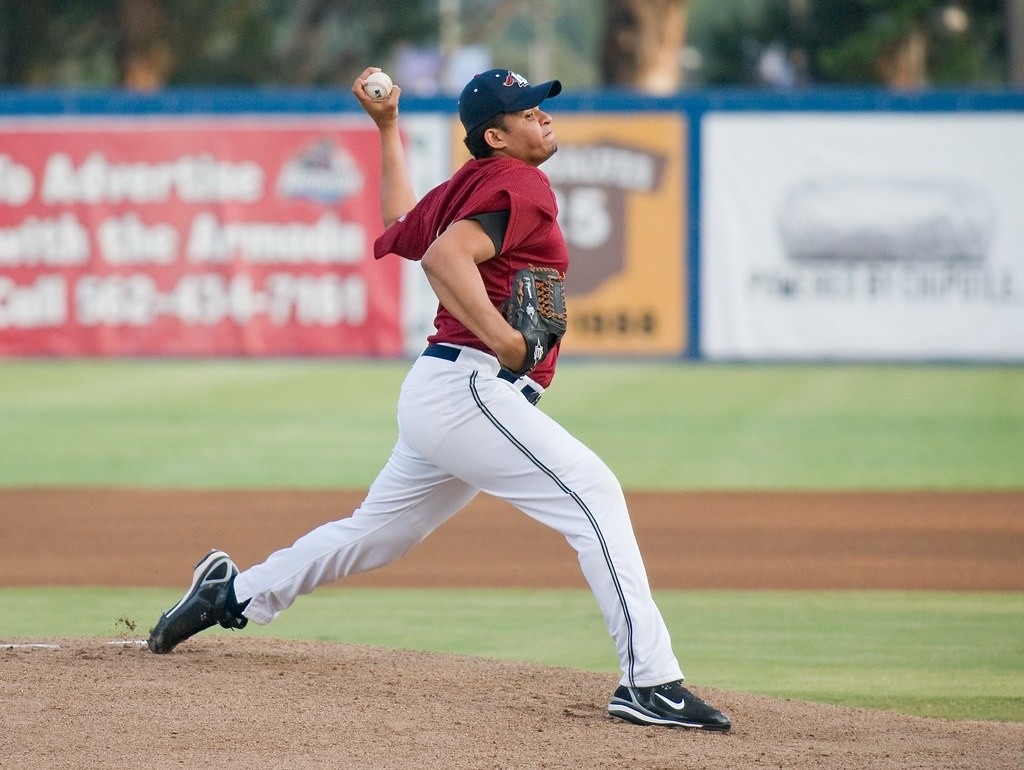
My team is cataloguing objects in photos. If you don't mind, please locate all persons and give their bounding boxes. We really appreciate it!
[148,66,732,733]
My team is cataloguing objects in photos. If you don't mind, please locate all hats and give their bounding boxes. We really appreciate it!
[459,69,562,136]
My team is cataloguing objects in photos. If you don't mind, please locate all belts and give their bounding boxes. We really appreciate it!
[420,344,542,406]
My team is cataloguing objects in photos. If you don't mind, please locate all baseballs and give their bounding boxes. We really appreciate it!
[363,71,393,100]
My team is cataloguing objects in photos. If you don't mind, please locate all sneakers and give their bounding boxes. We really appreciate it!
[147,548,249,654]
[607,678,732,731]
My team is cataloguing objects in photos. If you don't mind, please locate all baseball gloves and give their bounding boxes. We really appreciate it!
[494,265,569,379]
